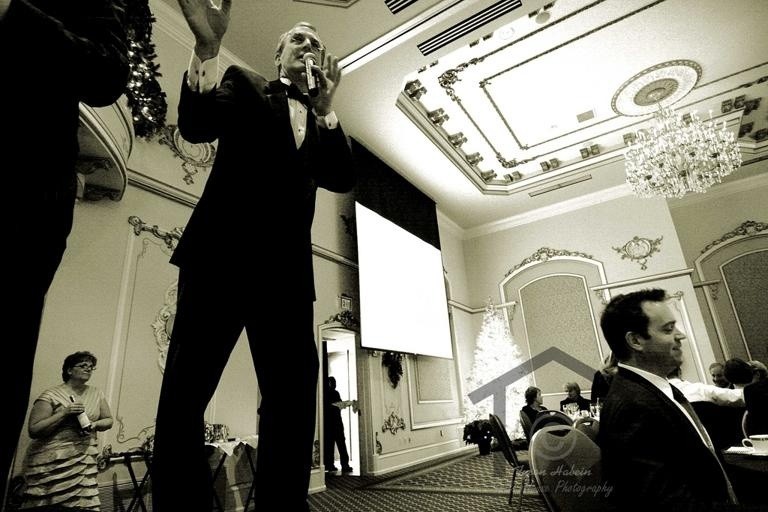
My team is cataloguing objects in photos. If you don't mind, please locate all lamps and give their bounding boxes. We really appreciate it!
[610,61,743,201]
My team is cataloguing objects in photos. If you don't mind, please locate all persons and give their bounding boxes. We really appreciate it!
[523,386,550,427]
[663,363,748,447]
[598,289,744,509]
[147,0,352,510]
[589,350,620,417]
[328,375,353,471]
[726,357,768,435]
[709,363,744,390]
[1,1,152,510]
[560,382,592,415]
[22,352,113,511]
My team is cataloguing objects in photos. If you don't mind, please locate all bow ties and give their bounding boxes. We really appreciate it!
[287,82,309,105]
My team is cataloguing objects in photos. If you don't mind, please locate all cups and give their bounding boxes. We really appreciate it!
[562,402,580,419]
[741,434,768,453]
[589,402,600,419]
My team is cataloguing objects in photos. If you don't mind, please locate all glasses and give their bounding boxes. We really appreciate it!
[290,32,323,53]
[74,363,96,370]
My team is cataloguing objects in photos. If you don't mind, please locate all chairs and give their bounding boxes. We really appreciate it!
[490,400,720,512]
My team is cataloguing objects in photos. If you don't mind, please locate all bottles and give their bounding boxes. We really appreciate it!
[69,395,92,433]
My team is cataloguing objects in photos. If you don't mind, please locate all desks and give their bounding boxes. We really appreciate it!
[721,444,768,473]
[204,434,260,511]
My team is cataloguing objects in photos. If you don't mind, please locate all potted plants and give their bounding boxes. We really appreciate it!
[463,420,494,455]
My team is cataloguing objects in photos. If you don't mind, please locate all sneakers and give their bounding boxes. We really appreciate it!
[342,467,353,473]
[325,466,338,471]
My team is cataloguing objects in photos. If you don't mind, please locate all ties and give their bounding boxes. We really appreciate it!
[670,383,739,506]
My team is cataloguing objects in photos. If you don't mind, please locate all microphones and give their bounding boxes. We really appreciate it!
[302,53,320,99]
